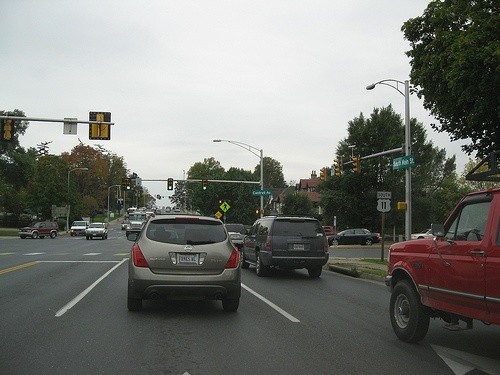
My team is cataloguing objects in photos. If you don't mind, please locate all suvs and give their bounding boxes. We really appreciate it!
[19,221,59,239]
[126,213,148,237]
[385,151,500,344]
[240,214,329,277]
[85,222,108,240]
[322,226,337,237]
[125,213,241,312]
[45,216,74,230]
[70,221,89,236]
[224,224,247,244]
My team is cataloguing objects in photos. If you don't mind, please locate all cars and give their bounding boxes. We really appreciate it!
[124,207,180,220]
[411,229,437,239]
[325,228,381,246]
[121,220,128,230]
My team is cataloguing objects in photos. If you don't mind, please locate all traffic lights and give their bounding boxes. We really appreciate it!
[168,179,173,191]
[320,167,326,182]
[202,179,208,191]
[352,157,359,173]
[334,159,340,177]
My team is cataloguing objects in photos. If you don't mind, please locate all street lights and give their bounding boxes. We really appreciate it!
[67,168,130,221]
[366,79,411,241]
[213,140,263,217]
